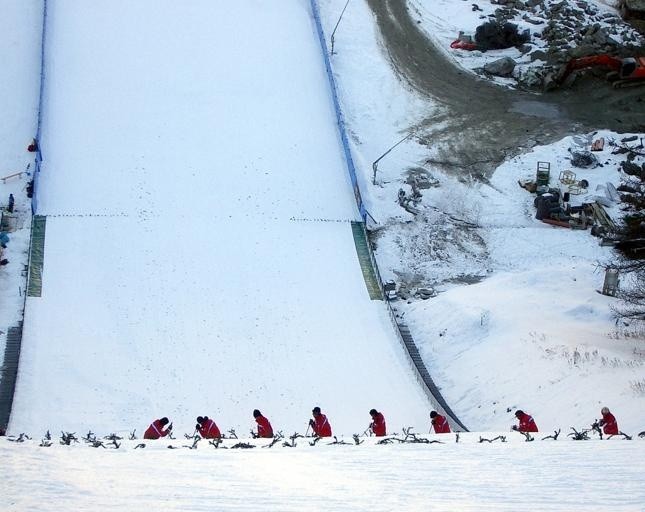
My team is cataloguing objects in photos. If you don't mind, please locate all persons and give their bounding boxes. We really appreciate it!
[253,410,273,438]
[8,193,14,213]
[513,410,539,432]
[308,407,331,437]
[430,411,450,433]
[144,417,172,440]
[195,416,221,439]
[599,408,619,434]
[369,409,386,436]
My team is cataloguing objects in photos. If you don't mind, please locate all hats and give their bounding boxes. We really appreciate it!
[430,411,437,418]
[314,407,320,414]
[197,416,203,422]
[253,410,261,417]
[369,409,377,415]
[515,410,523,416]
[601,407,609,414]
[161,417,168,425]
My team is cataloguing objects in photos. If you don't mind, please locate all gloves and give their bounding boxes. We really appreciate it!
[196,424,201,430]
[431,420,435,424]
[168,423,172,430]
[512,424,517,430]
[310,419,313,425]
